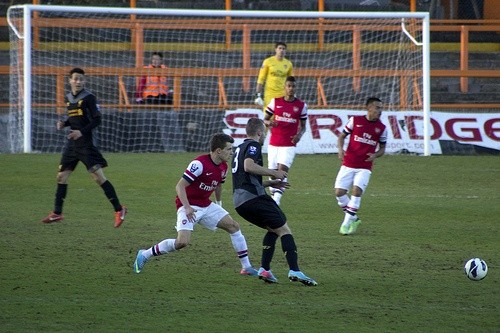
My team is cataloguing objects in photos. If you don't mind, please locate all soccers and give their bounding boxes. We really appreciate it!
[464,257,489,281]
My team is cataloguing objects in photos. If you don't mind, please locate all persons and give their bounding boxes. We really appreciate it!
[262,76,308,207]
[255,41,295,137]
[134,134,260,275]
[334,97,388,235]
[135,52,173,104]
[231,119,318,286]
[41,67,128,228]
